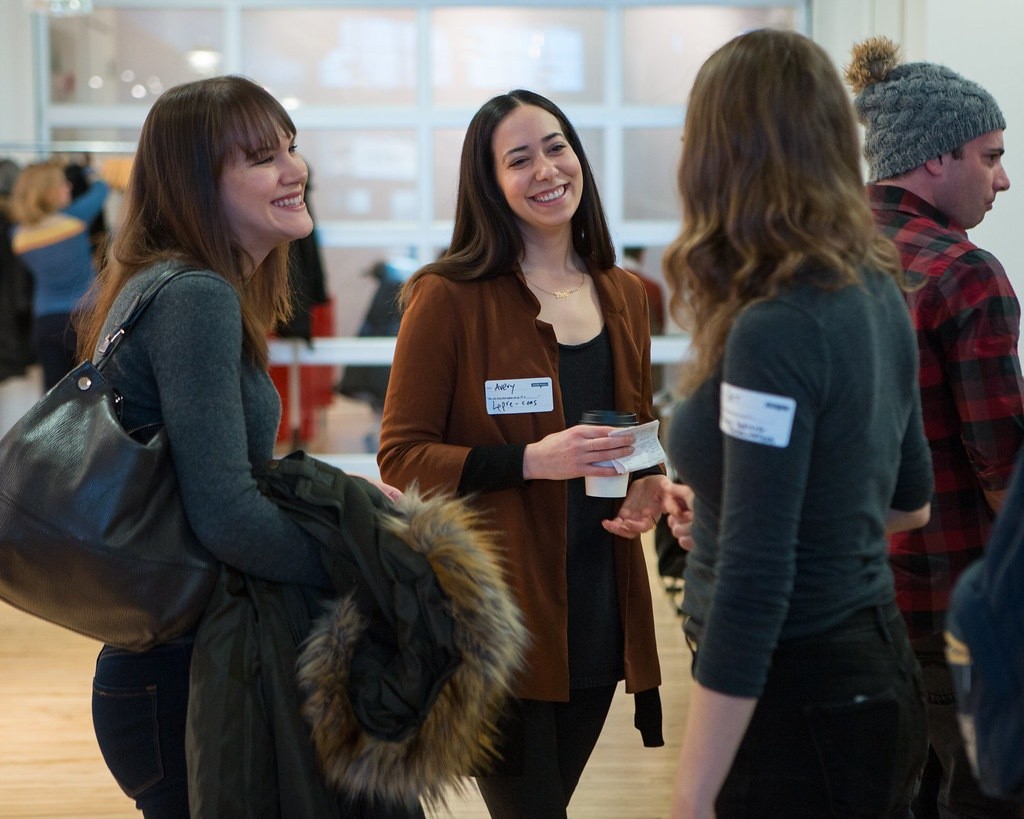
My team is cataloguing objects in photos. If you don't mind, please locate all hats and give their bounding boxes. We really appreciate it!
[844,35,1008,182]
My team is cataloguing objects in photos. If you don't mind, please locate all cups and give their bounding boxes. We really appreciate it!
[580,410,639,498]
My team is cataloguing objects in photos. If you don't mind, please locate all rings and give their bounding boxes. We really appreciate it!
[642,514,657,529]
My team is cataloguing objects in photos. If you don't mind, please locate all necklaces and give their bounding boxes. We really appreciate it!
[522,273,586,299]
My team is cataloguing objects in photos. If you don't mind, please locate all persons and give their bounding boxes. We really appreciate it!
[267,157,335,457]
[661,28,936,819]
[377,90,673,819]
[92,74,336,819]
[0,156,127,399]
[620,245,663,396]
[846,34,1024,819]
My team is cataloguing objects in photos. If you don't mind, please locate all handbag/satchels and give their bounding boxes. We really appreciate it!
[0,266,241,653]
